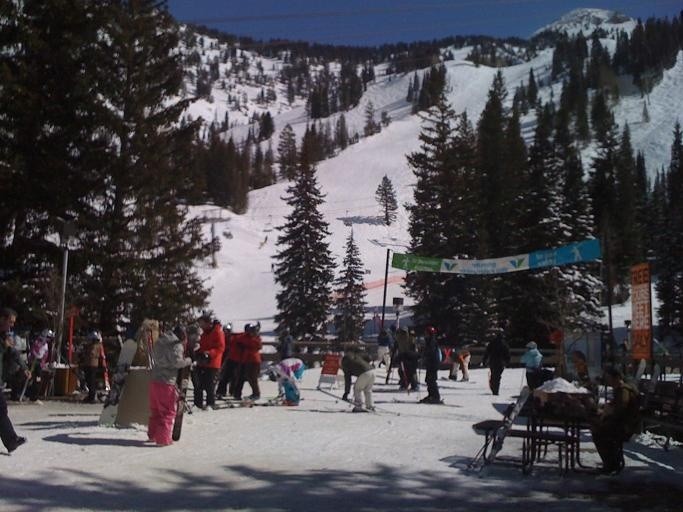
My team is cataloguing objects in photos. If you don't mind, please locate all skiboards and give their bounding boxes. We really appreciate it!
[186,383,305,409]
[371,376,476,407]
[310,405,400,417]
[97,331,112,391]
[172,321,198,441]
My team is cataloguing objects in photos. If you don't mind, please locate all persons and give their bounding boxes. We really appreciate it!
[418,325,445,402]
[520,340,543,390]
[146,324,194,446]
[482,327,512,396]
[269,357,304,405]
[336,349,374,412]
[589,360,642,472]
[376,322,472,390]
[566,350,600,413]
[0,309,32,453]
[6,314,261,407]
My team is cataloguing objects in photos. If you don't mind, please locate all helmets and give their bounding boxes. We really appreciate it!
[425,326,435,333]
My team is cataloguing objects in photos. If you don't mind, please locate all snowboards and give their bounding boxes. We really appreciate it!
[477,386,532,478]
[643,364,661,407]
[631,358,647,389]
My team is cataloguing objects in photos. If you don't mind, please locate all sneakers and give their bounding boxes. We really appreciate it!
[5,437,26,453]
[421,396,439,401]
[408,387,419,392]
[283,400,298,406]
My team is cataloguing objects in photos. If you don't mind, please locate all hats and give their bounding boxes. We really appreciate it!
[174,325,185,337]
[525,342,538,349]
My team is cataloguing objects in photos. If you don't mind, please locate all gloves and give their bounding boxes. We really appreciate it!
[342,392,348,401]
